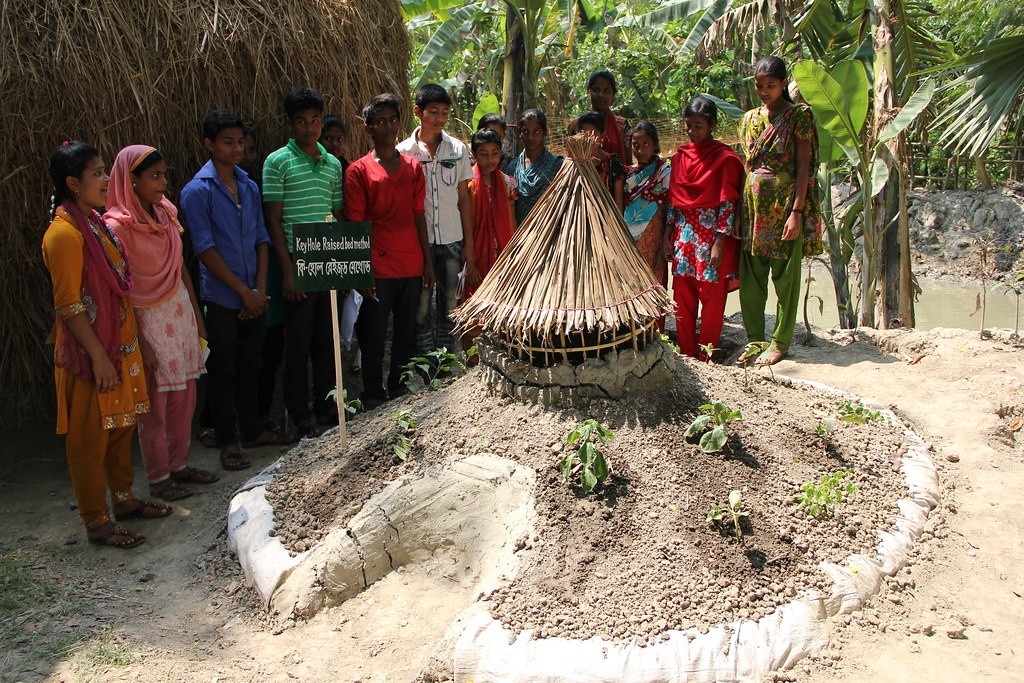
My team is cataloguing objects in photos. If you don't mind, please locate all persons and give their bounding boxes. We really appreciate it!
[180,110,269,469]
[42,141,173,547]
[623,121,669,288]
[469,113,515,171]
[104,144,220,500]
[462,128,517,368]
[578,114,625,217]
[663,98,746,362]
[394,84,475,385]
[735,58,823,366]
[345,94,436,409]
[505,109,564,226]
[263,91,360,439]
[566,71,632,166]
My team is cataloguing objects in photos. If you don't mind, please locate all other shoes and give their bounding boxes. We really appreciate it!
[288,419,321,435]
[315,406,349,423]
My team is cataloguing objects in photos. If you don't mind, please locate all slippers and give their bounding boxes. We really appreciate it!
[114,500,173,520]
[199,427,218,447]
[220,447,252,470]
[169,467,220,484]
[149,483,193,499]
[735,342,762,365]
[250,423,298,445]
[754,346,788,367]
[87,522,145,548]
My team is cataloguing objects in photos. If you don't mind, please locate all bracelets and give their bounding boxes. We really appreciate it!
[792,208,803,213]
